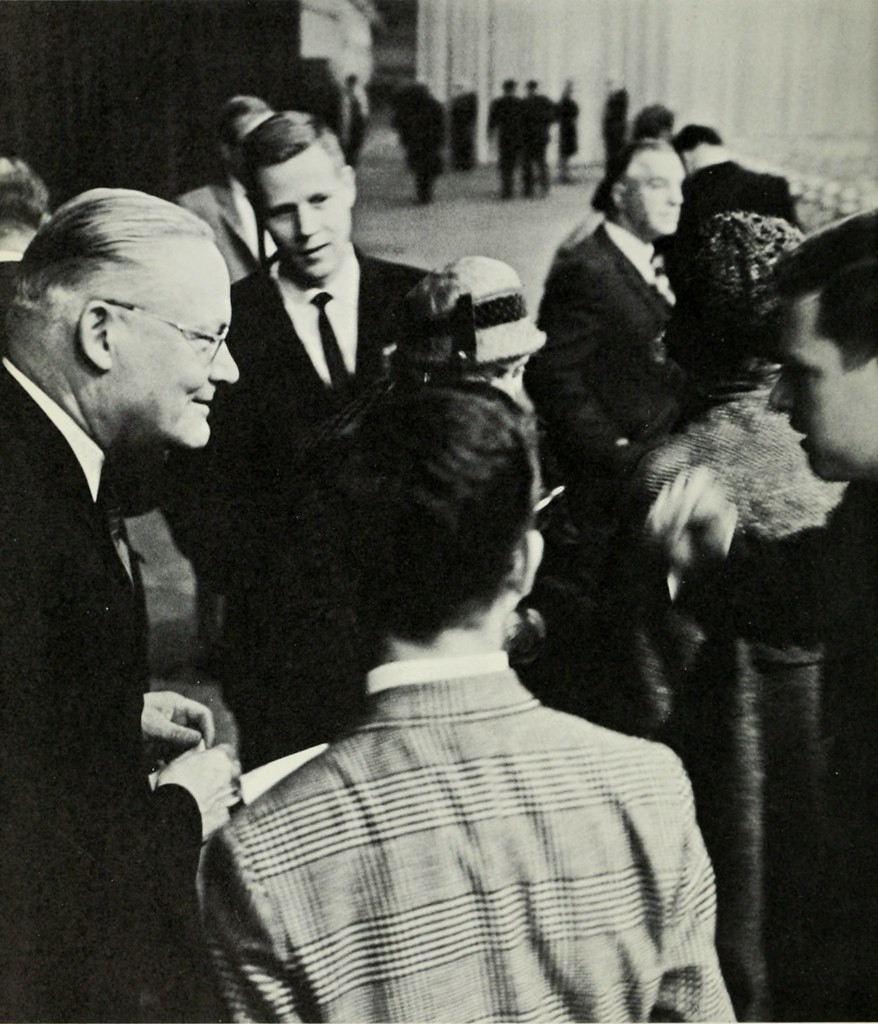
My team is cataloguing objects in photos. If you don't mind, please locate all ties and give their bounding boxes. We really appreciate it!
[310,293,351,395]
[245,193,266,264]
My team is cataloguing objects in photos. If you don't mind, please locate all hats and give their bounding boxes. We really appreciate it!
[382,256,547,365]
[677,209,804,330]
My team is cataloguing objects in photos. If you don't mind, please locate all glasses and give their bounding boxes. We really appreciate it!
[91,297,228,368]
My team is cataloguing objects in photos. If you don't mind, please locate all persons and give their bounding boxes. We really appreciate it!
[0,73,878,1024]
